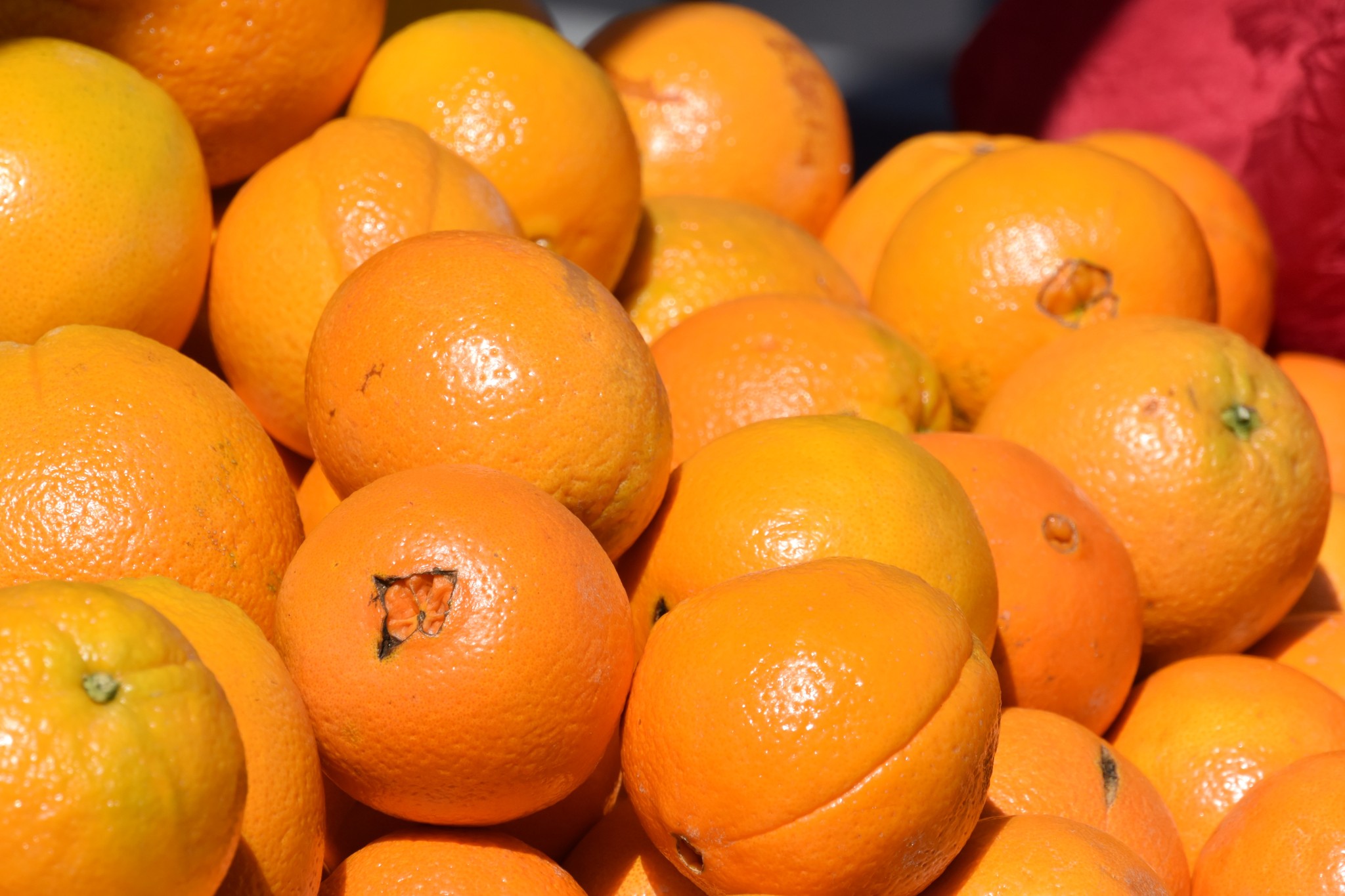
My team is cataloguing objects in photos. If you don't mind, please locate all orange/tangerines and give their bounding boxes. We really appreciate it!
[0,0,1345,896]
